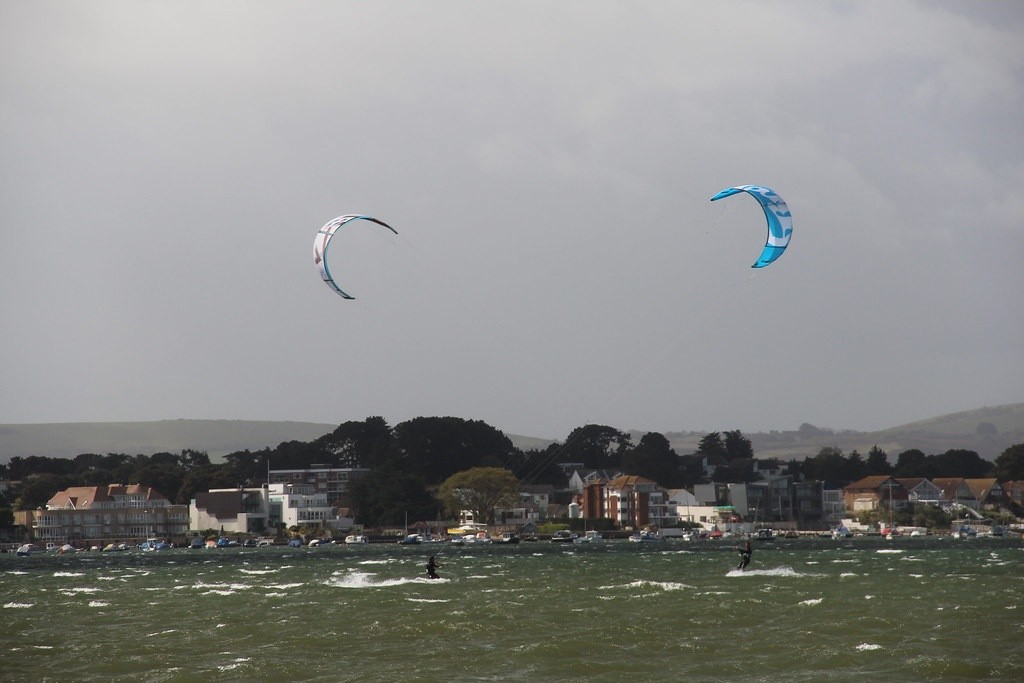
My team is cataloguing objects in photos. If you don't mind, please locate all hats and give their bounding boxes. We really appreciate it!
[430,557,434,560]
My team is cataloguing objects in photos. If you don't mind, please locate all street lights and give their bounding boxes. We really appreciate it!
[144,511,148,542]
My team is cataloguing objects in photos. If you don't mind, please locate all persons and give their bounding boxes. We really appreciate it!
[426,556,441,578]
[738,542,752,570]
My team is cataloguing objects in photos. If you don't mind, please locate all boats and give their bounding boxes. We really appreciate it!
[575,531,603,544]
[452,536,489,548]
[525,536,539,542]
[987,527,1011,536]
[308,540,320,547]
[911,530,920,539]
[289,541,300,547]
[629,533,659,543]
[60,536,256,551]
[398,534,423,545]
[332,537,368,546]
[954,489,968,539]
[16,547,35,557]
[753,530,775,540]
[489,531,520,545]
[831,528,854,539]
[551,530,578,543]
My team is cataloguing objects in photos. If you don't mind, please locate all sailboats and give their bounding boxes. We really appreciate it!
[783,486,799,539]
[681,485,698,543]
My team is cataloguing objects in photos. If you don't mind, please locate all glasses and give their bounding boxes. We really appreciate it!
[745,545,748,546]
